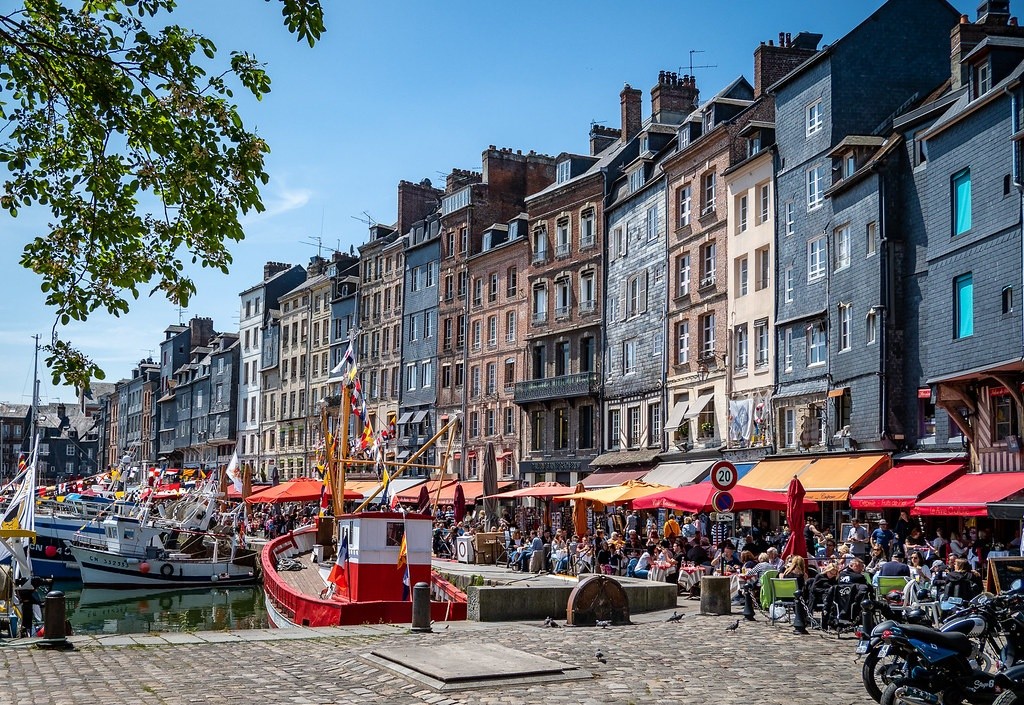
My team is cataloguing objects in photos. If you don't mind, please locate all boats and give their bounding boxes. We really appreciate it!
[258,325,467,632]
[28,491,143,542]
[0,333,85,637]
[31,391,263,592]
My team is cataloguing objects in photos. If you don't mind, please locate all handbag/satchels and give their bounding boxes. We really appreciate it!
[669,532,676,542]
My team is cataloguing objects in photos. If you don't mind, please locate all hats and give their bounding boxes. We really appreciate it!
[894,552,903,558]
[930,560,943,571]
[878,519,888,524]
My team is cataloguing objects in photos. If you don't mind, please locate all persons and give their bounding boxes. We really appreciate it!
[431,505,981,634]
[208,499,427,544]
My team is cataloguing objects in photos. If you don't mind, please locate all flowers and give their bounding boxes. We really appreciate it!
[701,422,711,429]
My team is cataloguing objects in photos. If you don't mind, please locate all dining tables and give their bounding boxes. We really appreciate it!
[648,563,677,581]
[677,567,705,600]
[986,550,1019,559]
[714,570,757,599]
[907,547,930,561]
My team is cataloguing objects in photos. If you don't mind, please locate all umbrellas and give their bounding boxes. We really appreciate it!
[454,484,466,526]
[480,480,671,541]
[633,481,818,514]
[418,484,430,516]
[782,476,807,561]
[245,475,364,504]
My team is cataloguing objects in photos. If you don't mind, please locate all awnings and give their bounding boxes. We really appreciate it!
[224,483,271,499]
[579,453,1024,516]
[342,480,515,506]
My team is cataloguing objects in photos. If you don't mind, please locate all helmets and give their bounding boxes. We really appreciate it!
[907,609,932,626]
[916,588,934,602]
[886,590,904,606]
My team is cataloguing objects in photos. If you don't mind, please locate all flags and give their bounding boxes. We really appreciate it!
[128,466,213,479]
[226,450,242,494]
[36,472,113,496]
[313,337,412,600]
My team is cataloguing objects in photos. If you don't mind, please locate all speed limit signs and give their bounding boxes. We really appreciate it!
[711,461,738,491]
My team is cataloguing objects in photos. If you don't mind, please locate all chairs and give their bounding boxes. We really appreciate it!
[430,515,1024,633]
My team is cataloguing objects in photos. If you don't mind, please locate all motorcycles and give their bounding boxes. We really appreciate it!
[854,607,1005,703]
[870,591,1024,705]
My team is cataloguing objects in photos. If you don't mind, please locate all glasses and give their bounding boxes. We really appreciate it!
[874,549,879,551]
[830,571,835,576]
[827,544,832,547]
[911,557,917,559]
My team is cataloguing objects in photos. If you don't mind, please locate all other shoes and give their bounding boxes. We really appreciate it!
[513,561,519,564]
[510,564,518,566]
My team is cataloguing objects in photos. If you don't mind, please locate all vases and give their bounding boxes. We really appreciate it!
[703,429,709,436]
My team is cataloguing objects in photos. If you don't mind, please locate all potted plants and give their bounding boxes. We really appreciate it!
[679,425,688,440]
